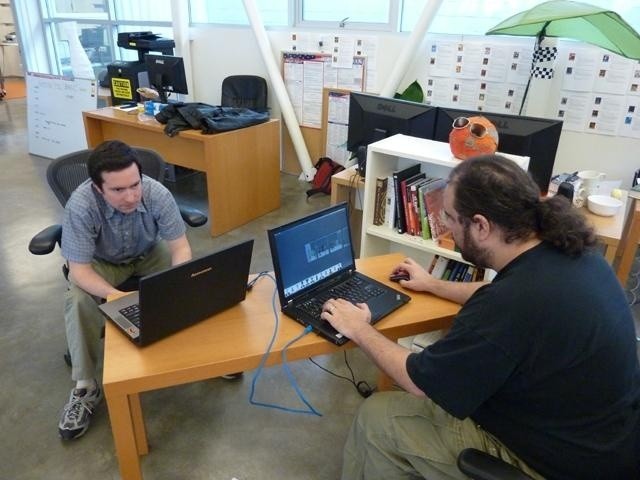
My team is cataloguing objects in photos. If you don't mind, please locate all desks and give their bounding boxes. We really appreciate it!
[102,253,465,480]
[82,101,281,237]
[617,180,640,287]
[330,163,628,266]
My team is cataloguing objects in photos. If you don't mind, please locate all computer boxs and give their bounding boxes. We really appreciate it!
[159,155,202,182]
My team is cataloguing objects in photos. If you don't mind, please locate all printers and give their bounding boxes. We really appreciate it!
[108,31,175,107]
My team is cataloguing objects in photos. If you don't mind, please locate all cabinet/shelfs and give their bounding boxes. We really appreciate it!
[0,3,17,46]
[360,133,531,284]
[0,46,24,78]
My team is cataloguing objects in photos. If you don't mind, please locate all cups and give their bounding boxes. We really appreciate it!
[577,170,606,207]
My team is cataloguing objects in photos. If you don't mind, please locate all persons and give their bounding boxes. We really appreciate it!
[318,154,640,480]
[57,141,244,441]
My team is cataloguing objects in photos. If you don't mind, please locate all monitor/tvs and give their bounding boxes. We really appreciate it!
[433,107,564,196]
[144,53,188,105]
[347,92,438,183]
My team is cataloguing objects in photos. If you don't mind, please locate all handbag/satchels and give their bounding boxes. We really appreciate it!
[305,157,345,198]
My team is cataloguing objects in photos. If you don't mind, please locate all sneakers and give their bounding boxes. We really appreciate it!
[58,383,104,441]
[222,371,243,380]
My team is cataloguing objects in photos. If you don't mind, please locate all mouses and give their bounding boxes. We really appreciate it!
[389,270,409,281]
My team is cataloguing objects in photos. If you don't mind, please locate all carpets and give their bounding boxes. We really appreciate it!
[1,80,26,100]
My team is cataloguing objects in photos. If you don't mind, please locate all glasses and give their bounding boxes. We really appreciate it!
[452,117,498,147]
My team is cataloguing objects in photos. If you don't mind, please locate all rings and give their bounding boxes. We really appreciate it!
[329,305,336,313]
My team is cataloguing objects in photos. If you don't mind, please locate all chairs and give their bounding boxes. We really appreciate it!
[220,75,268,114]
[29,146,208,366]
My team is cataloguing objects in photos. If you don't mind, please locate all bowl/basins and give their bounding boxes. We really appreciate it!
[587,195,623,216]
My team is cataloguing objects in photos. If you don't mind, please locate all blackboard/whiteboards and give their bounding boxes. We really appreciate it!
[25,71,97,160]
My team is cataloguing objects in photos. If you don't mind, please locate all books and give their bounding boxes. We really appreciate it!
[429,254,486,284]
[398,328,451,353]
[372,161,462,252]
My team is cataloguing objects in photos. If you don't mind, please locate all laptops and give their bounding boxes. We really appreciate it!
[267,201,411,347]
[97,239,253,346]
